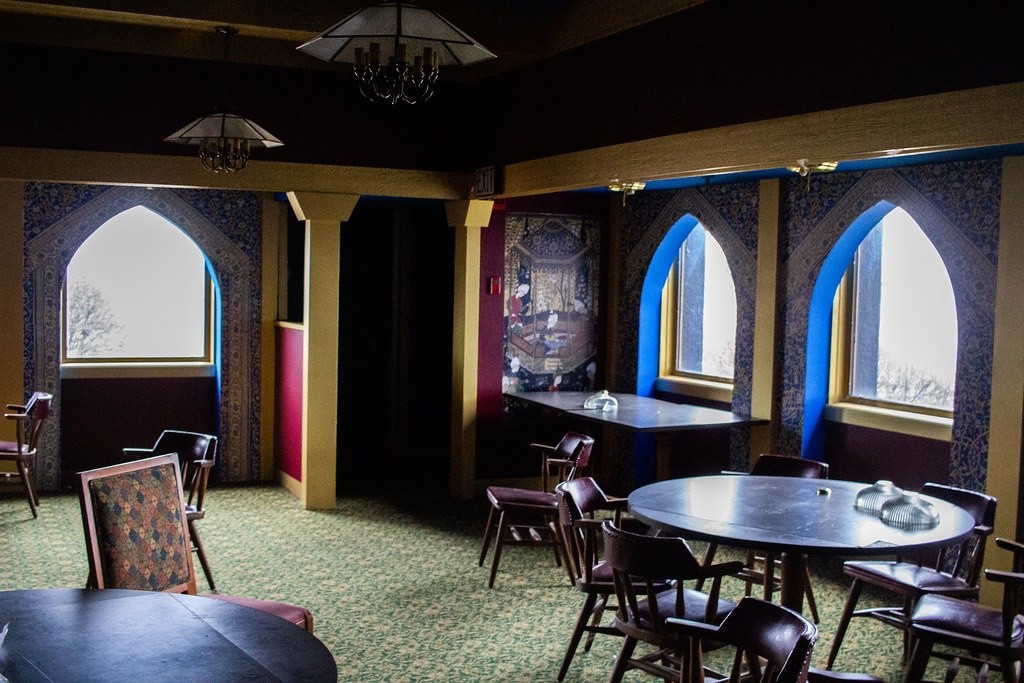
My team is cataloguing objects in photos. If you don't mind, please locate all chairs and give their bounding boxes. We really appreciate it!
[555,476,677,683]
[0,392,53,518]
[479,432,594,588]
[824,482,998,671]
[76,453,313,634]
[85,430,218,590]
[601,520,743,683]
[694,454,828,624]
[901,537,1024,683]
[664,596,819,683]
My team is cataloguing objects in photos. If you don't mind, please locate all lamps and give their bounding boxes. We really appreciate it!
[164,26,285,174]
[296,0,498,105]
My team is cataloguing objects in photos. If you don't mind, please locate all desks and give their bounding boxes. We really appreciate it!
[504,392,770,488]
[0,590,338,683]
[627,475,975,615]
